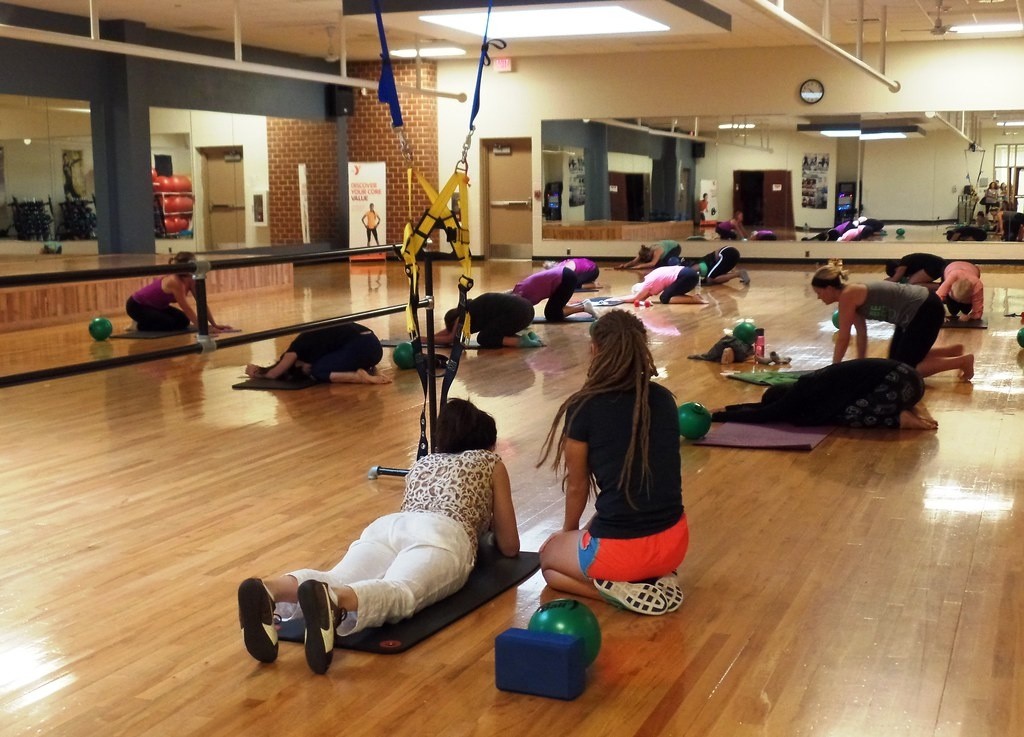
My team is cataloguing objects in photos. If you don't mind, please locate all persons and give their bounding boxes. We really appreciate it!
[124,252,234,335]
[245,323,392,384]
[618,239,684,269]
[709,358,938,430]
[681,246,750,285]
[605,266,709,304]
[713,211,777,242]
[811,258,974,381]
[802,217,885,241]
[699,193,709,221]
[421,293,548,347]
[883,253,983,321]
[945,180,1024,243]
[543,258,600,289]
[505,266,599,321]
[535,310,689,616]
[61,153,83,201]
[238,397,521,674]
[361,203,381,246]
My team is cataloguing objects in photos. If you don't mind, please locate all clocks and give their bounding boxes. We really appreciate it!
[800,79,825,104]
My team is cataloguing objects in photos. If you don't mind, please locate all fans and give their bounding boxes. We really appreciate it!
[900,0,958,35]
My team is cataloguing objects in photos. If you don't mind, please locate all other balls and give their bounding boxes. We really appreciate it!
[1016,328,1024,347]
[527,597,603,665]
[897,228,905,235]
[88,317,113,341]
[699,261,708,277]
[151,168,193,233]
[677,402,713,440]
[733,322,757,345]
[956,224,963,229]
[832,309,841,330]
[392,341,414,369]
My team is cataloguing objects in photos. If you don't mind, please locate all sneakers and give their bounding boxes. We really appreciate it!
[655,568,683,612]
[593,578,668,616]
[297,579,347,676]
[238,577,278,663]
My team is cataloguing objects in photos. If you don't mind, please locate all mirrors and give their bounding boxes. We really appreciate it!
[540,108,1024,242]
